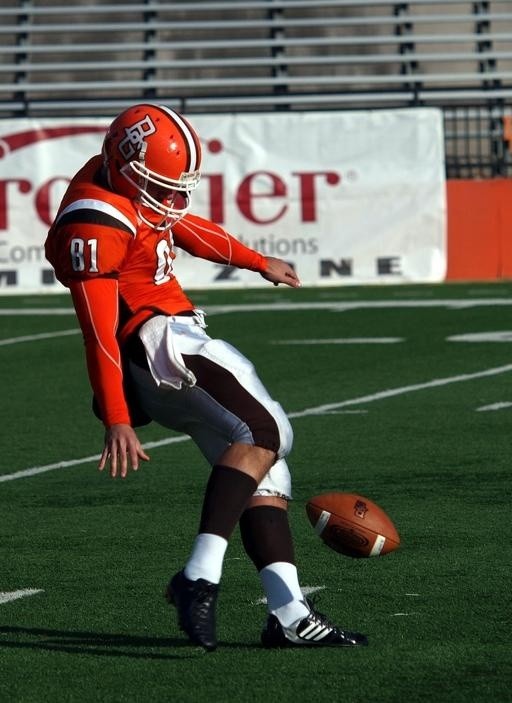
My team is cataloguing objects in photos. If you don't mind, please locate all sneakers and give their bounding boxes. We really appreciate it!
[165,570,219,651]
[261,593,368,648]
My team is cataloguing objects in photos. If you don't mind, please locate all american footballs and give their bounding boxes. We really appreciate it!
[305,494,401,558]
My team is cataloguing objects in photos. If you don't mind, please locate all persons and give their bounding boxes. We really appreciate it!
[44,102,369,653]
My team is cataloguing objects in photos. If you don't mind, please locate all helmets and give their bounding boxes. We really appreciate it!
[102,103,201,231]
